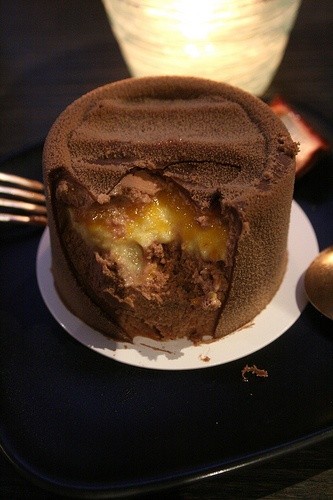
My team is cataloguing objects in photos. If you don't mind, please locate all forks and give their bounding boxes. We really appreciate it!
[0,172,48,224]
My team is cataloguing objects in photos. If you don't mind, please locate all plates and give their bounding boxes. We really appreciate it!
[36,199,320,371]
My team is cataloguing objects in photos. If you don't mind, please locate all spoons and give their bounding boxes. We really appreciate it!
[304,245,333,319]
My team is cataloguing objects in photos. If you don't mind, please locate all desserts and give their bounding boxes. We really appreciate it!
[41,75,298,343]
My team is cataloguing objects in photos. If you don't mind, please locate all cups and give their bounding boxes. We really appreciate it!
[101,1,303,98]
